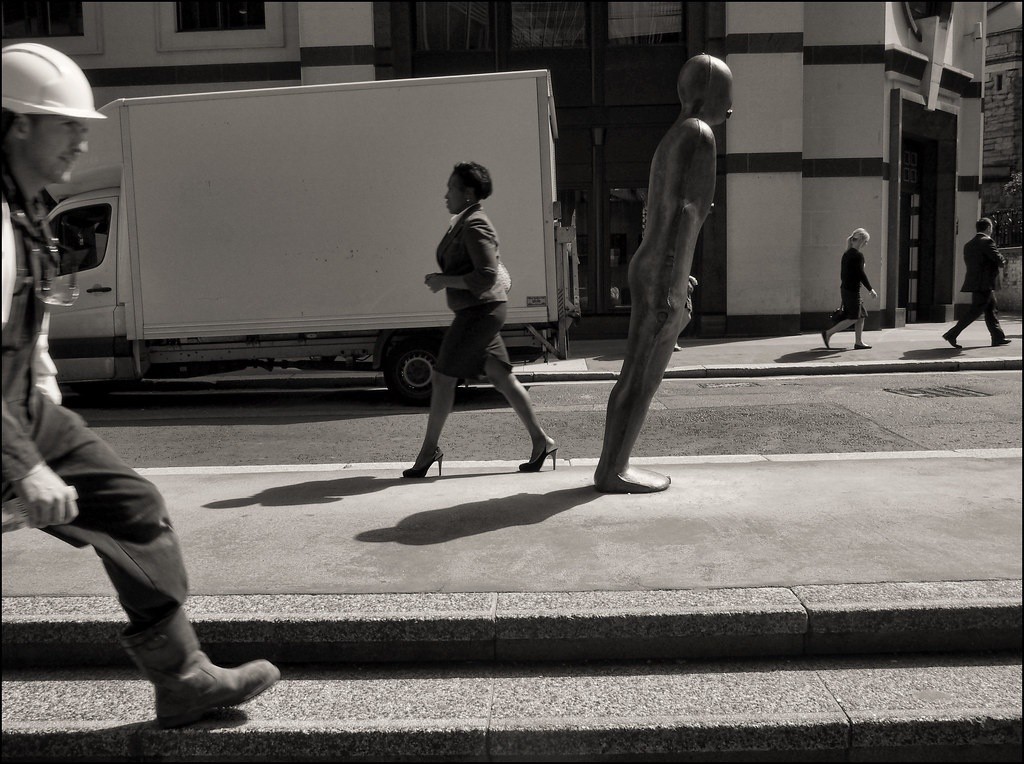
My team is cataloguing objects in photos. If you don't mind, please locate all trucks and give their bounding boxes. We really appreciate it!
[40,69,582,405]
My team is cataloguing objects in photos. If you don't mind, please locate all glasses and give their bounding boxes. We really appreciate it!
[29,242,79,312]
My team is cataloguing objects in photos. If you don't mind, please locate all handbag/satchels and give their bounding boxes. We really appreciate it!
[496,261,512,294]
[830,303,855,329]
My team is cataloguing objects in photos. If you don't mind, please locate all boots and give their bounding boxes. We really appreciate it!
[118,604,281,727]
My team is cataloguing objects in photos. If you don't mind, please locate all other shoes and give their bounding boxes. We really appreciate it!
[854,344,872,349]
[992,340,1011,347]
[822,330,829,349]
[943,332,962,349]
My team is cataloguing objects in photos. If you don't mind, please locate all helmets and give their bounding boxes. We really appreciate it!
[2,42,108,120]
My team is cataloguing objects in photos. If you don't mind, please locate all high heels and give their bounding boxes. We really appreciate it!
[403,447,443,478]
[519,436,558,472]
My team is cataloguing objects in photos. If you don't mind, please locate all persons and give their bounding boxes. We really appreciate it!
[942,218,1011,349]
[593,51,733,495]
[0,42,279,725]
[822,227,877,350]
[403,162,557,481]
